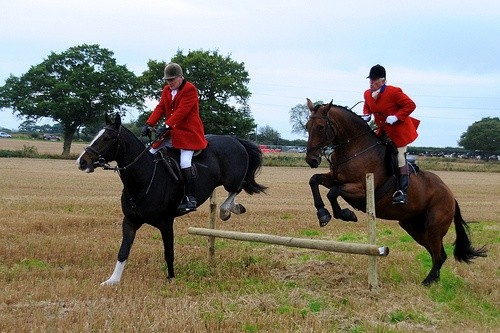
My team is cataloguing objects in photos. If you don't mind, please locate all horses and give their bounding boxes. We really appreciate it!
[76,111,269,287]
[305,98,488,287]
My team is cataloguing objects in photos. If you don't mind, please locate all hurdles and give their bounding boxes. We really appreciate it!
[188,174,389,291]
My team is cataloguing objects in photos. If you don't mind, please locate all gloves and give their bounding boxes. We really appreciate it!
[155,124,166,140]
[142,123,151,136]
[386,115,397,125]
[361,114,370,120]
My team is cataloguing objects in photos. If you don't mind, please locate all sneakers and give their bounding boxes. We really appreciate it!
[186,196,197,211]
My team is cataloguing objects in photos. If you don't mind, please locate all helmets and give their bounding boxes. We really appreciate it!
[162,63,183,79]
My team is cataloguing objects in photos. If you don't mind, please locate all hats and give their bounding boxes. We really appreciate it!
[366,64,386,80]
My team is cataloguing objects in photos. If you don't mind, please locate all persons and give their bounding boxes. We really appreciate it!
[361,64,419,202]
[141,63,208,209]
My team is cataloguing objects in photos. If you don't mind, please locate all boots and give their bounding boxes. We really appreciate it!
[393,174,408,205]
